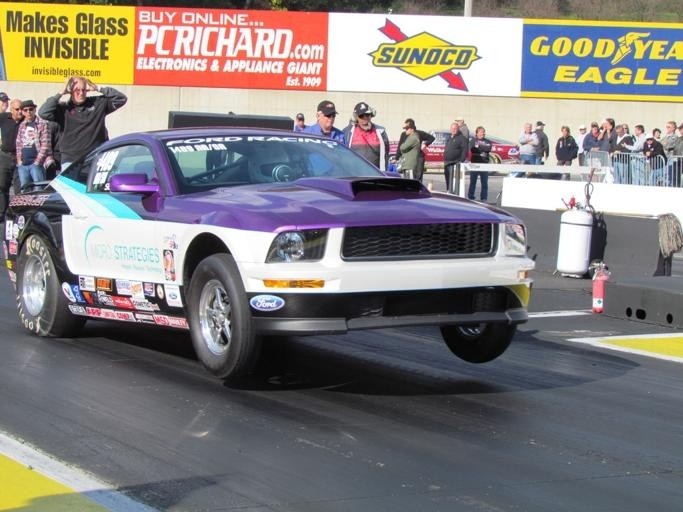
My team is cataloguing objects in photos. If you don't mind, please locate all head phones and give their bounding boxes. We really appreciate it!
[351,107,376,122]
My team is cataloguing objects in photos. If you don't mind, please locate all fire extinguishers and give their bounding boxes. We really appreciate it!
[555,195,595,281]
[588,260,611,314]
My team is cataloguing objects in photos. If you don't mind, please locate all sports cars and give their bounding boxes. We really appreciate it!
[2,127,536,378]
[381,129,520,176]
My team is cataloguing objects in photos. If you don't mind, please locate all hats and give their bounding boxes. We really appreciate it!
[20,100,37,109]
[317,101,338,114]
[354,102,372,114]
[0,92,10,102]
[296,113,304,120]
[403,124,416,130]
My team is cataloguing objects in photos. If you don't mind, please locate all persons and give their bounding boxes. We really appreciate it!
[443,116,493,201]
[342,101,391,175]
[518,119,551,178]
[556,126,578,180]
[577,118,683,189]
[0,72,128,219]
[304,100,345,146]
[396,118,436,182]
[295,112,309,132]
[246,141,291,183]
